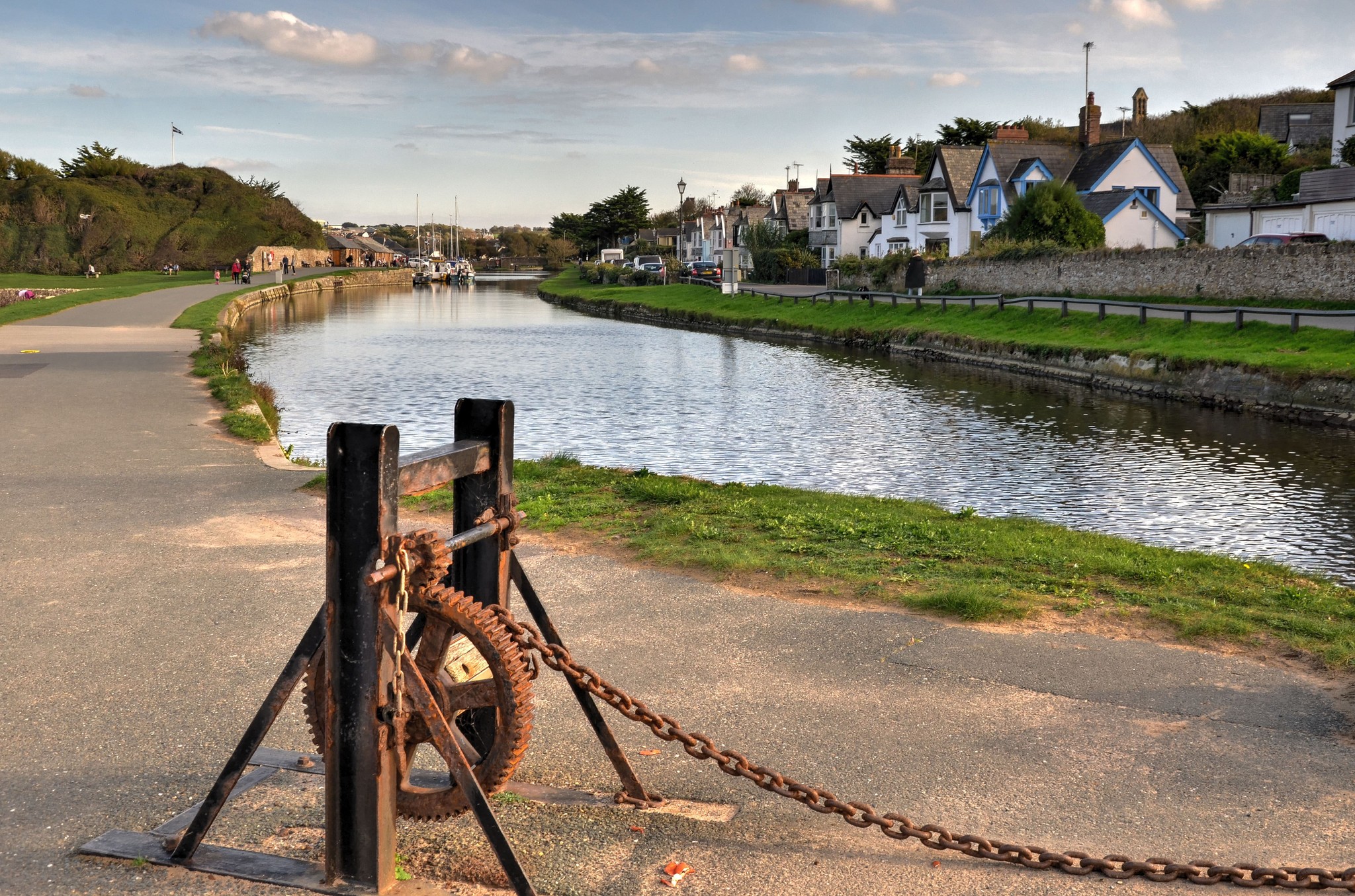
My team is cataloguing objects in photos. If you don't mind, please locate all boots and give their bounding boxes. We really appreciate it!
[235,280,236,284]
[291,273,295,275]
[217,281,219,284]
[238,280,238,284]
[216,281,217,284]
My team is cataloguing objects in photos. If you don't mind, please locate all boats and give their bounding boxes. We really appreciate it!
[449,251,476,281]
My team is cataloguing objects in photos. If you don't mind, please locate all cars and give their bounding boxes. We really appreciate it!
[404,258,432,267]
[682,261,721,284]
[594,260,601,265]
[623,262,633,267]
[637,263,664,278]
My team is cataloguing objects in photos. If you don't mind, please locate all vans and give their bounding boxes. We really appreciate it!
[393,254,401,259]
[632,255,662,270]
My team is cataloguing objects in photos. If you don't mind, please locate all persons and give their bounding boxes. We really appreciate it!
[435,262,440,272]
[164,263,179,275]
[214,270,220,285]
[15,290,35,301]
[242,259,251,284]
[88,264,95,278]
[578,258,583,265]
[291,255,295,275]
[232,258,241,284]
[364,252,409,268]
[327,255,335,267]
[349,253,354,267]
[267,253,272,271]
[283,255,289,275]
[905,249,925,301]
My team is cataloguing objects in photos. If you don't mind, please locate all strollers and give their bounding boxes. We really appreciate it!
[241,268,251,284]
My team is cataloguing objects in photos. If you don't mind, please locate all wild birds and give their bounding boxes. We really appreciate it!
[80,214,91,219]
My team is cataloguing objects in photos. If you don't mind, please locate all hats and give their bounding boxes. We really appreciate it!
[235,259,239,263]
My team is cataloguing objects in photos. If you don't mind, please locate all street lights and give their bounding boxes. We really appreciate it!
[623,236,628,251]
[564,230,566,243]
[618,235,620,247]
[652,227,656,249]
[634,232,637,251]
[581,244,583,258]
[677,177,687,269]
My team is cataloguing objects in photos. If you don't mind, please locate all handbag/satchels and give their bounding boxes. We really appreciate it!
[922,261,932,275]
[232,273,234,280]
[269,262,271,265]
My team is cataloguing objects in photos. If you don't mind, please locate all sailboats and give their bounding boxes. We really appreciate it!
[410,193,461,283]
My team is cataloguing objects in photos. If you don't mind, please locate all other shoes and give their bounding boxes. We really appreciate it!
[270,270,272,271]
[287,273,288,274]
[284,273,286,274]
[268,271,270,272]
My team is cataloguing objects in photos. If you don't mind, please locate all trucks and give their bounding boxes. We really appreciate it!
[600,249,623,265]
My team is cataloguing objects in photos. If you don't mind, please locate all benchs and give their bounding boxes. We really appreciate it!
[303,261,310,267]
[85,272,100,278]
[157,266,181,273]
[326,260,387,266]
[317,261,324,267]
[212,266,229,273]
[280,262,291,268]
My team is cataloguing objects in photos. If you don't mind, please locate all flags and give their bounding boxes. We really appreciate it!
[172,126,183,134]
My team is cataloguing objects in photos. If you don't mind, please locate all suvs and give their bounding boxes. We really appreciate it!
[604,258,630,267]
[1233,233,1328,245]
